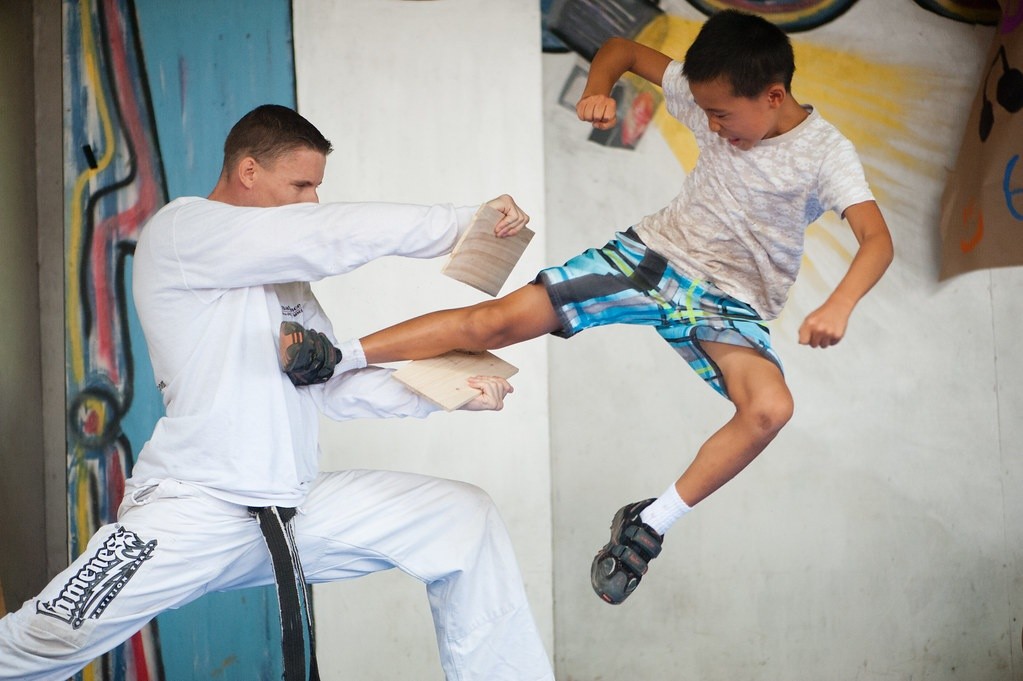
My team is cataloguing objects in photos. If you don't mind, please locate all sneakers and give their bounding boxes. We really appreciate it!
[591,496,664,605]
[279,320,342,390]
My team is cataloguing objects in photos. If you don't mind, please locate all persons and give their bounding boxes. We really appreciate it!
[2,101,554,681]
[279,9,898,603]
